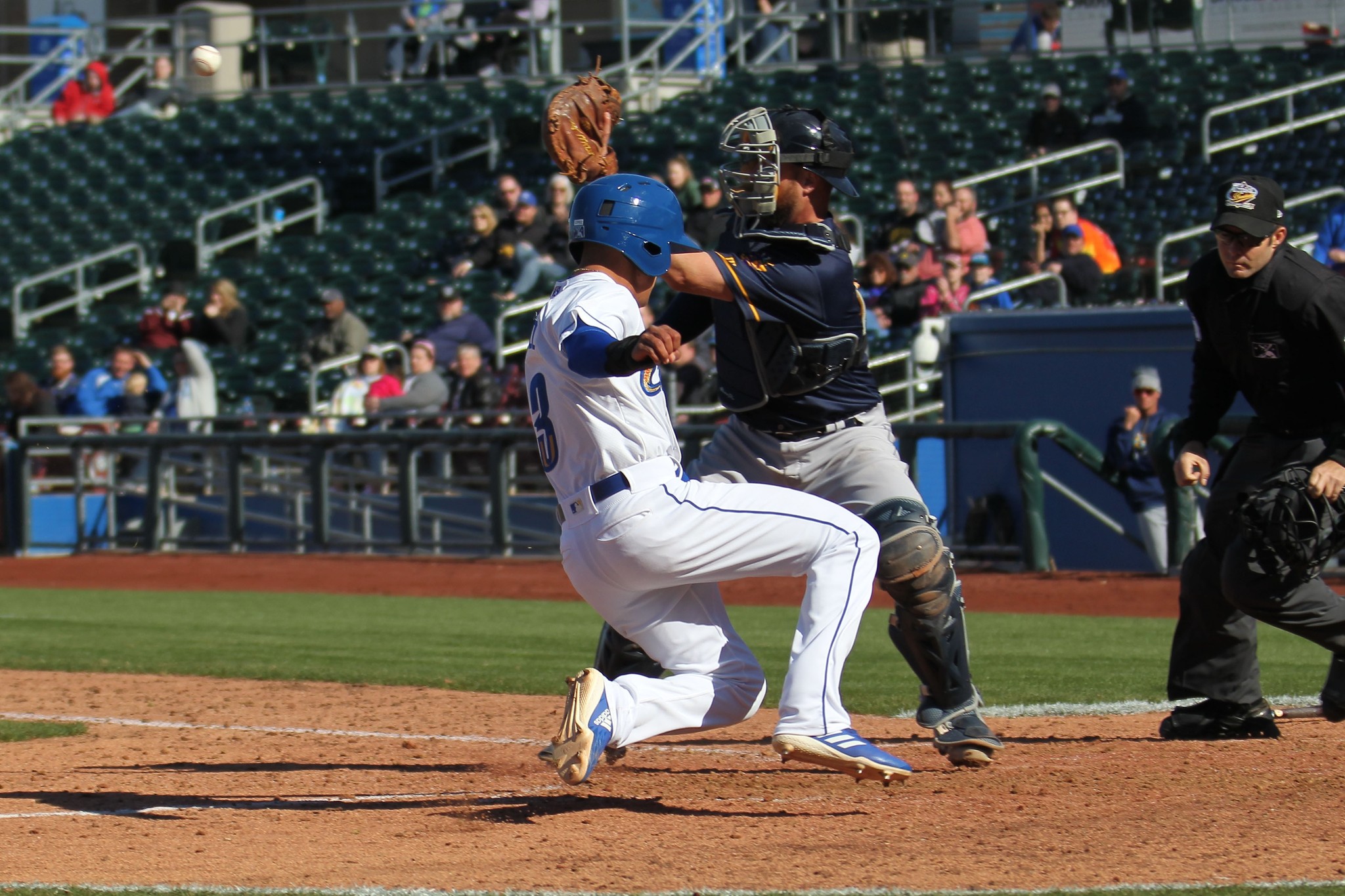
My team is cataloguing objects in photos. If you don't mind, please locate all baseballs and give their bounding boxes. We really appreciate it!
[189,45,222,77]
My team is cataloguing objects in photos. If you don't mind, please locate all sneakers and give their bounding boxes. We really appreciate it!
[1159,697,1273,737]
[1320,654,1345,722]
[772,728,912,787]
[551,667,613,785]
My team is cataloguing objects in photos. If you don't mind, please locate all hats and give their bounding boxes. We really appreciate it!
[1060,225,1082,239]
[1131,367,1162,394]
[518,190,537,207]
[364,345,380,358]
[1211,175,1284,238]
[896,253,917,268]
[969,254,989,264]
[321,288,341,303]
[942,254,961,268]
[413,341,434,356]
[439,285,459,302]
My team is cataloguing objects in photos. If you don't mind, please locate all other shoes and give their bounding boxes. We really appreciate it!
[539,745,554,762]
[933,727,992,767]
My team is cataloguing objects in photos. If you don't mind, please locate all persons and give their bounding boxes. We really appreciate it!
[1157,177,1345,737]
[1010,7,1061,53]
[297,171,575,492]
[1024,198,1122,305]
[0,333,217,528]
[524,175,914,786]
[546,54,1003,764]
[1107,367,1206,573]
[836,178,1014,384]
[198,279,249,348]
[138,283,193,349]
[385,0,528,80]
[115,55,188,122]
[51,61,115,123]
[1314,205,1345,275]
[1085,70,1146,160]
[1020,84,1082,151]
[649,156,732,246]
[755,0,791,61]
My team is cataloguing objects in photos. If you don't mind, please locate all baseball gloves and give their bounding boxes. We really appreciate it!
[545,73,621,180]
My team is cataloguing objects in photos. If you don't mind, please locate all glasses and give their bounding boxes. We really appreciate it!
[1213,227,1272,248]
[1134,388,1155,395]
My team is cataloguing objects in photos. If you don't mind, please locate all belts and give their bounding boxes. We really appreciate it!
[760,415,861,442]
[556,465,689,525]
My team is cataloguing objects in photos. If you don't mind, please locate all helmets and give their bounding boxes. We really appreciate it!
[769,108,860,198]
[568,173,703,276]
